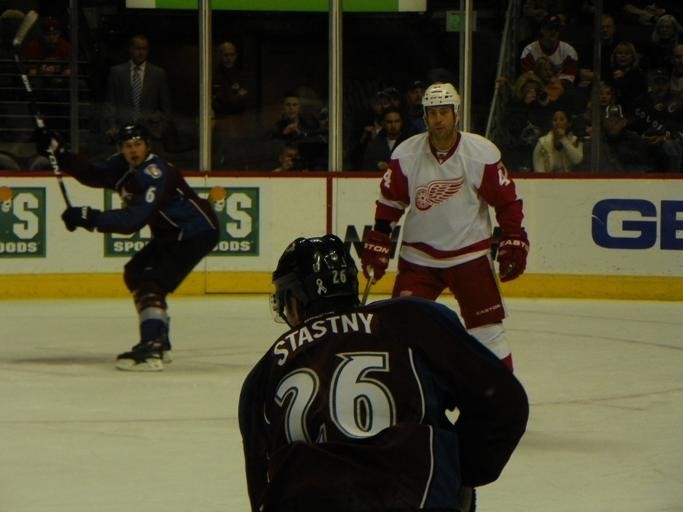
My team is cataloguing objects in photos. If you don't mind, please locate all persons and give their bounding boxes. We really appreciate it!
[36,123,219,370]
[489,2,682,179]
[0,2,426,178]
[361,83,530,376]
[237,233,530,512]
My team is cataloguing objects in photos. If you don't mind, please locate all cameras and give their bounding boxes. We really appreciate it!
[535,88,547,100]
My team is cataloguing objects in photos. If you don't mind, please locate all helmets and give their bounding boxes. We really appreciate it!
[269,234,358,323]
[117,123,150,146]
[423,81,461,130]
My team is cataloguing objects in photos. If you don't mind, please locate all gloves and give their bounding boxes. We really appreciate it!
[61,206,100,232]
[491,227,530,283]
[361,229,392,285]
[34,126,68,161]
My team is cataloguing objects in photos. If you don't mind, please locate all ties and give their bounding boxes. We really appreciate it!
[132,67,142,124]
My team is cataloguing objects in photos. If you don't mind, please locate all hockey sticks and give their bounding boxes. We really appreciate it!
[10,10,72,209]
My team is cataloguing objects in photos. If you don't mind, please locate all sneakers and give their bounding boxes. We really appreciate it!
[118,335,171,358]
